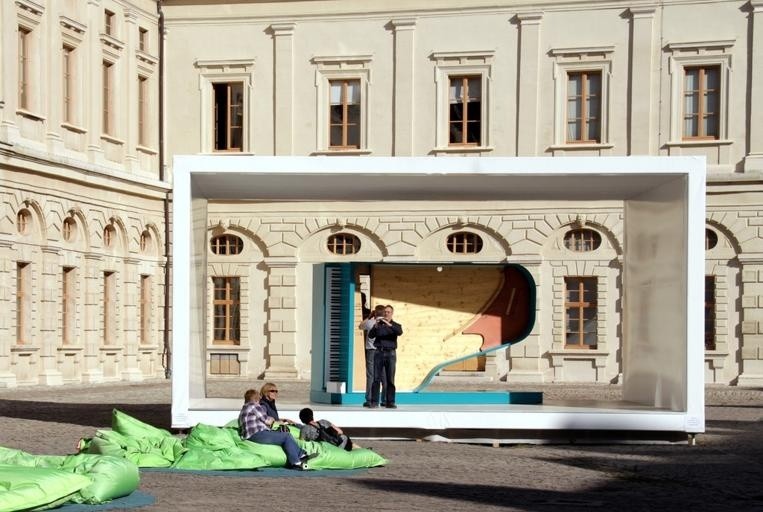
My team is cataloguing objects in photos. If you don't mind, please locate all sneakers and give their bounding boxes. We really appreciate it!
[291,453,318,470]
[363,401,397,408]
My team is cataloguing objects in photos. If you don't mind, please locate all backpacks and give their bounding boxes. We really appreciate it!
[309,422,343,447]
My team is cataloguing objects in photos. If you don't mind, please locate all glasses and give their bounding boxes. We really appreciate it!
[268,389,278,393]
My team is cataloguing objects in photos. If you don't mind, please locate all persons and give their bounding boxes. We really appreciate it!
[362,293,370,320]
[300,408,361,451]
[359,305,403,408]
[238,383,318,471]
